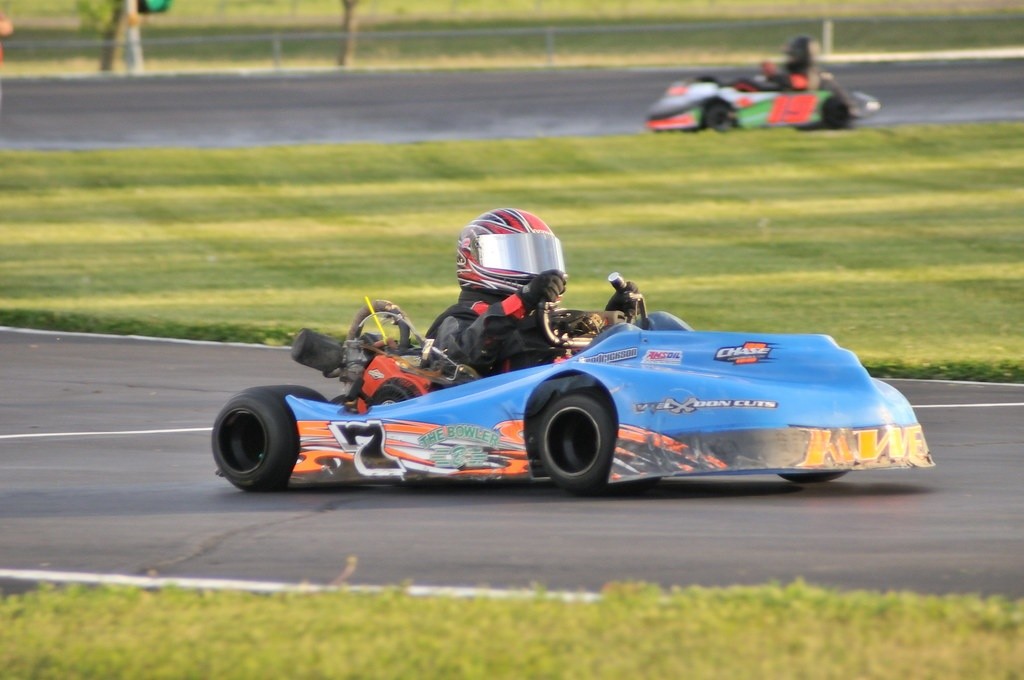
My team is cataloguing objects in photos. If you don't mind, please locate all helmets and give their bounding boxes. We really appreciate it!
[783,36,820,72]
[457,208,569,295]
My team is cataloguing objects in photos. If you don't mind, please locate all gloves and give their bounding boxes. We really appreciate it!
[605,282,641,311]
[515,268,567,316]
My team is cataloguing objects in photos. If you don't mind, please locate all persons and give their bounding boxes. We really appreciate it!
[762,36,821,91]
[420,208,646,385]
